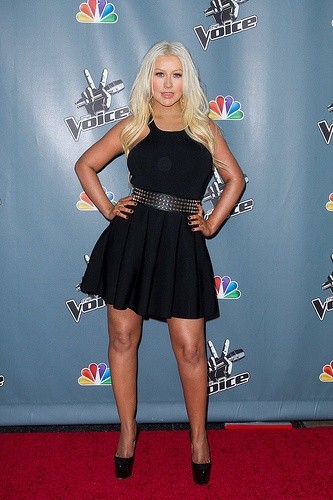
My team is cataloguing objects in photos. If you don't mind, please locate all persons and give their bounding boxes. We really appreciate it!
[74,41,245,485]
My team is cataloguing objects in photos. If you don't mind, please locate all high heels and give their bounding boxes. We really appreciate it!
[189,431,212,487]
[113,428,139,478]
